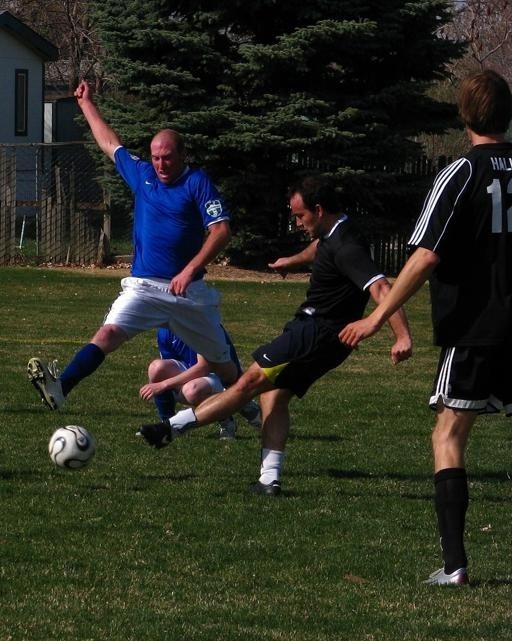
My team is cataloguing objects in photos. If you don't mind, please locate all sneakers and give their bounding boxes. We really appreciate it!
[220,418,236,442]
[252,480,281,496]
[135,417,172,450]
[26,357,66,411]
[240,400,261,424]
[421,567,468,587]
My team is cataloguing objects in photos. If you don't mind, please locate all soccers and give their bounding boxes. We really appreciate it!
[48,425,95,470]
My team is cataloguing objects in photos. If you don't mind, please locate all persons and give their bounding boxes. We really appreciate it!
[334,68,512,588]
[25,78,262,438]
[138,326,240,440]
[133,174,415,496]
